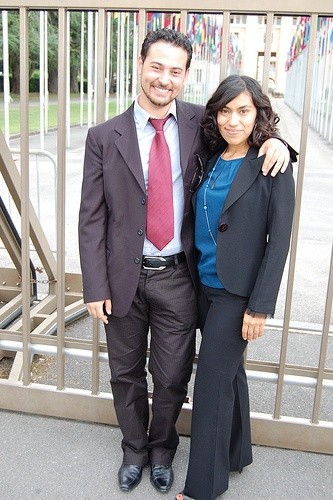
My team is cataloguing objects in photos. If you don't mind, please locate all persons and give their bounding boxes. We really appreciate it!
[175,74,296,500]
[77,27,298,492]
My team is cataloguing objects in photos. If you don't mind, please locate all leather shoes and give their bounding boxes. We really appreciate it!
[118,464,143,492]
[150,464,174,492]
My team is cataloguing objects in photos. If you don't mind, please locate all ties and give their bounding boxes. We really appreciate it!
[145,113,174,251]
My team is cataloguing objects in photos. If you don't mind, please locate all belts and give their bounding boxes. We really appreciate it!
[141,251,186,270]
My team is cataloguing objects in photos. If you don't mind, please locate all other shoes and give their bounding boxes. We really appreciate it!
[175,491,195,500]
